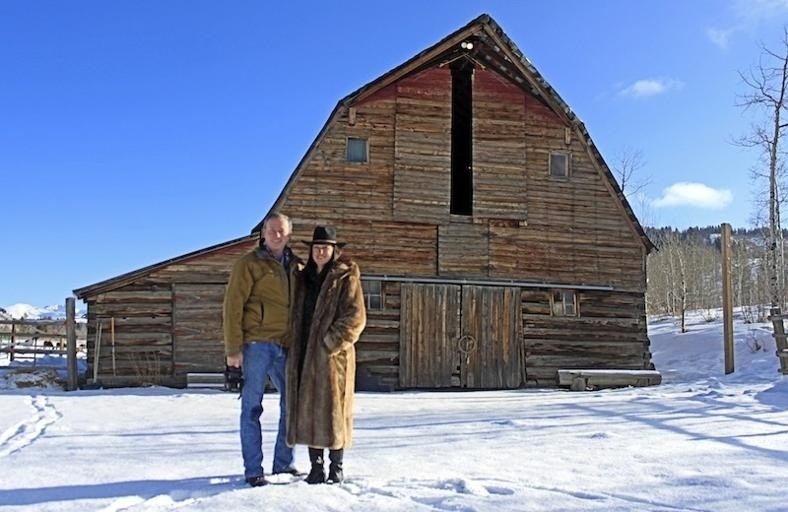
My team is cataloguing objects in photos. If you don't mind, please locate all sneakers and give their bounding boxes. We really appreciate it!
[273,468,306,476]
[246,476,268,486]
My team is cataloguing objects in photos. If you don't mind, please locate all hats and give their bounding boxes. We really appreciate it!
[301,225,346,248]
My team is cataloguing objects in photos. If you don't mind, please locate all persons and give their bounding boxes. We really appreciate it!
[283,225,367,484]
[221,212,305,486]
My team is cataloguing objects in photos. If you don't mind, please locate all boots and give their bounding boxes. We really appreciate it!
[304,447,325,484]
[327,449,344,485]
